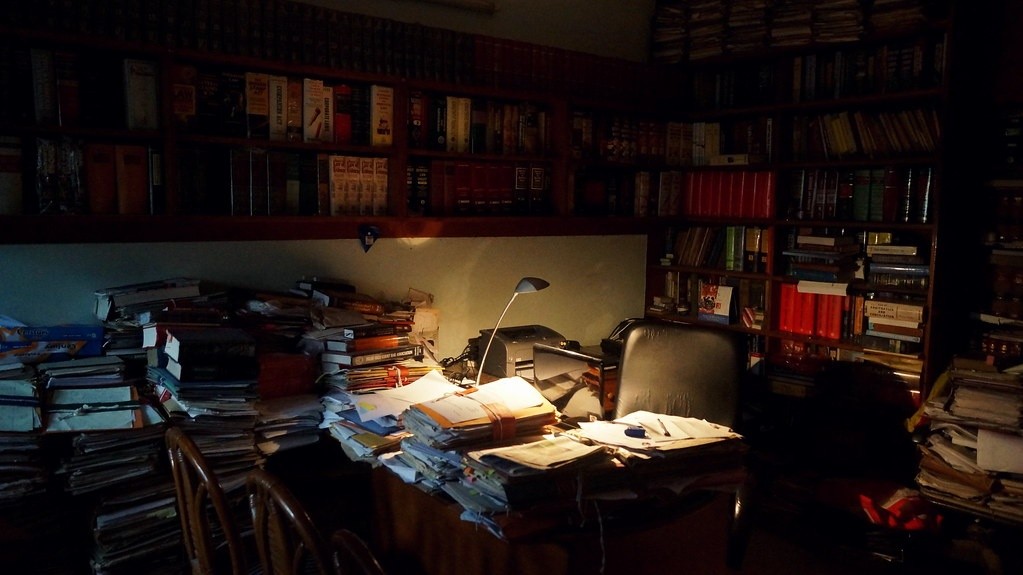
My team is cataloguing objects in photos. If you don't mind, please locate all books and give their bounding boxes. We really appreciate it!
[1,0,1023,574]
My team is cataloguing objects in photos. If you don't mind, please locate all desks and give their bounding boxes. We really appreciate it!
[337,343,752,575]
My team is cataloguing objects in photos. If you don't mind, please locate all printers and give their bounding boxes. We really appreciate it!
[477,325,567,379]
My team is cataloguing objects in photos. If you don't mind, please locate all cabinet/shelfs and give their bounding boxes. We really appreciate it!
[0,0,1023,536]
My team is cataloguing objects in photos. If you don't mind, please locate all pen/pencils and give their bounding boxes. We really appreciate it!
[657,419,671,437]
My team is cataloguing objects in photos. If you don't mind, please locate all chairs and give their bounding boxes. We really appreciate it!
[165,427,386,575]
[611,317,748,435]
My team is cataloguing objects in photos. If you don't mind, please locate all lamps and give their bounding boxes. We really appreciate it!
[475,277,550,388]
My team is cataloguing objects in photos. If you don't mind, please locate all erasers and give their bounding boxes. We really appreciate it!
[624,426,646,438]
